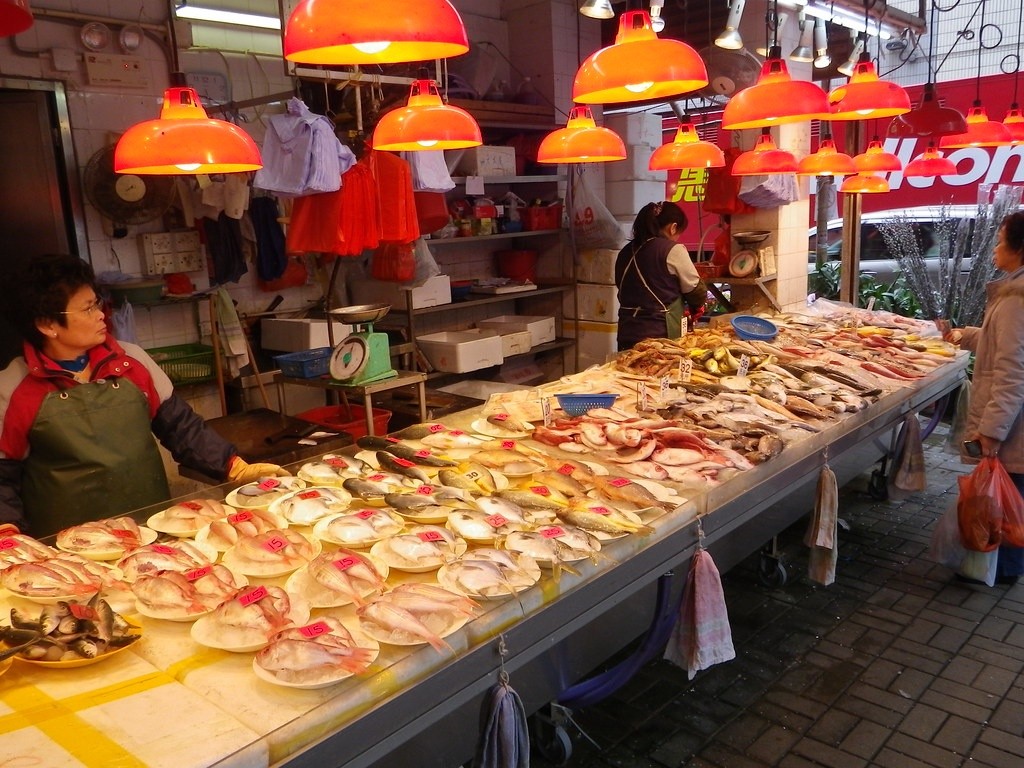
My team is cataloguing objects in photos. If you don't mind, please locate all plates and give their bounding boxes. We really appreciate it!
[0,417,668,689]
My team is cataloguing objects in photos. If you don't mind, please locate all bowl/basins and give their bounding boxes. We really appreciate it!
[102,279,167,304]
[451,280,473,299]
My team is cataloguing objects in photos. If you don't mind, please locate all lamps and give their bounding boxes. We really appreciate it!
[82,23,149,88]
[534,0,1024,195]
[283,0,469,64]
[372,66,483,151]
[115,0,264,174]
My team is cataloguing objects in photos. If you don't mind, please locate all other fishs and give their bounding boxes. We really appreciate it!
[301,424,497,512]
[754,310,957,381]
[447,438,679,583]
[215,585,296,632]
[0,535,124,595]
[356,582,485,658]
[486,408,756,487]
[57,517,143,550]
[236,477,349,524]
[328,509,469,564]
[636,341,882,465]
[256,616,379,675]
[165,499,226,528]
[307,547,391,608]
[447,548,544,616]
[237,529,314,563]
[0,583,142,659]
[117,541,240,614]
[207,508,280,545]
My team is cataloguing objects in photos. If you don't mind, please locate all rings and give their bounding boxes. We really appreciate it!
[994,451,998,455]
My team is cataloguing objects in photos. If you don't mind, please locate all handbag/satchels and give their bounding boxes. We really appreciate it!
[259,256,306,291]
[988,455,1024,549]
[929,496,998,587]
[958,455,1003,553]
[713,225,731,274]
[366,236,414,282]
[398,238,440,291]
[561,170,625,254]
[112,302,138,346]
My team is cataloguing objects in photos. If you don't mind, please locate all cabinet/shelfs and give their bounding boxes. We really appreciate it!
[349,116,578,421]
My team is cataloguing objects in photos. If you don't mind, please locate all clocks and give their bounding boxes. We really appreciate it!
[185,69,232,122]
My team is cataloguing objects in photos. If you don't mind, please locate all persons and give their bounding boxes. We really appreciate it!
[942,210,1024,588]
[614,201,708,352]
[0,250,292,537]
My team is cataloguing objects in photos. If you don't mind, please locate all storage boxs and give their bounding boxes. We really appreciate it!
[418,332,509,374]
[487,327,535,357]
[143,317,359,387]
[477,312,559,348]
[350,276,455,312]
[560,110,661,376]
[517,205,564,231]
[453,146,517,176]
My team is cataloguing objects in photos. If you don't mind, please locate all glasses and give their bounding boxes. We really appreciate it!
[58,298,105,317]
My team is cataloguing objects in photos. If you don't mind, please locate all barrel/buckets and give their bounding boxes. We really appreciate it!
[414,192,450,234]
[494,249,538,282]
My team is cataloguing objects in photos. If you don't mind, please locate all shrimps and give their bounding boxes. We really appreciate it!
[618,339,704,378]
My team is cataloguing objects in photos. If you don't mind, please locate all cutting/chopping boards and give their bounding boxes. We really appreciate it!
[204,408,353,467]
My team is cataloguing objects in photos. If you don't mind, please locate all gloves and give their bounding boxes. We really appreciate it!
[227,456,292,482]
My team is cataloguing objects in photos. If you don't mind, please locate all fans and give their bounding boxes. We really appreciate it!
[82,142,175,237]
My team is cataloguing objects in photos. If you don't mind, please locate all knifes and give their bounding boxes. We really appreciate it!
[264,424,316,444]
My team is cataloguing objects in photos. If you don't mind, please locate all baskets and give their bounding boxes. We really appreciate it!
[557,394,620,417]
[694,261,725,278]
[731,315,778,342]
[273,346,335,379]
[145,343,225,386]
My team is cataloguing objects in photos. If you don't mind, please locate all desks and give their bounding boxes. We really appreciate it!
[274,371,427,439]
